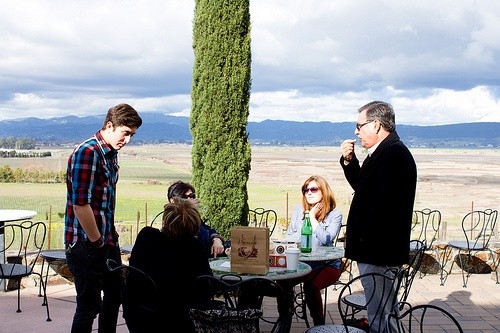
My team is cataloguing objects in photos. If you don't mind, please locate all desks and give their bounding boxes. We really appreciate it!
[207,257,313,281]
[0,210,38,291]
[299,246,345,261]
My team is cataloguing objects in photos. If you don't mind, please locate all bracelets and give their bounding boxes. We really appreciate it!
[91,235,104,247]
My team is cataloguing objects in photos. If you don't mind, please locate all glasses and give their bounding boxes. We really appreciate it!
[356,120,373,130]
[303,187,321,194]
[179,193,196,200]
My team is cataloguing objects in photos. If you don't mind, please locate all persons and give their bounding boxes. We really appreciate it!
[271,175,344,333]
[65,104,142,333]
[122,199,215,333]
[339,101,417,333]
[168,180,229,299]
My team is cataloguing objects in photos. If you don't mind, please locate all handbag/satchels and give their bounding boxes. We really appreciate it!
[230,228,269,276]
[189,309,263,333]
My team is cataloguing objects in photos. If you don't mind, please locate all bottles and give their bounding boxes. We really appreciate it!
[300,210,312,252]
[272,238,298,253]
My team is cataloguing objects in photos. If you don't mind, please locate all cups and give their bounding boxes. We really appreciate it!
[285,250,301,271]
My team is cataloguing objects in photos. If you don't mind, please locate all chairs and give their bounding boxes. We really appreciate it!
[0,208,500,333]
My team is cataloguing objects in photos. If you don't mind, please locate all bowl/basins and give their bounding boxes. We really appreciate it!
[268,253,286,266]
